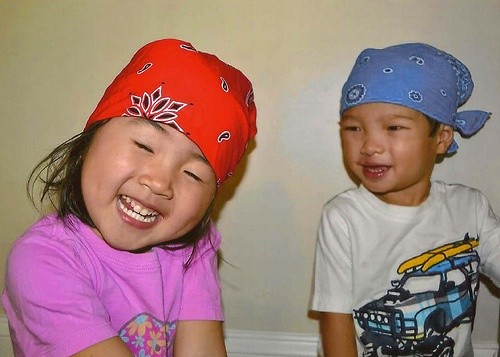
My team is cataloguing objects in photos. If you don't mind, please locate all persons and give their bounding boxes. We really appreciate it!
[308,40,500,357]
[0,38,258,357]
